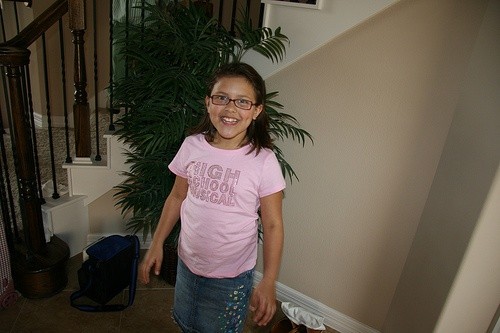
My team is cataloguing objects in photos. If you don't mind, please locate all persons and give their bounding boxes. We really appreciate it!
[138,62,287,333]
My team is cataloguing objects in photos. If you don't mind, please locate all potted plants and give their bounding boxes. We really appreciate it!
[102,0,313,286]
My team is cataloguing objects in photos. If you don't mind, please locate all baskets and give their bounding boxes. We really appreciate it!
[272,302,325,333]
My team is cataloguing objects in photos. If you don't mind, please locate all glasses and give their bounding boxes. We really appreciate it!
[208,95,257,110]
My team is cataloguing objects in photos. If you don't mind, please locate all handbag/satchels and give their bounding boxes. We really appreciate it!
[76,235,138,304]
